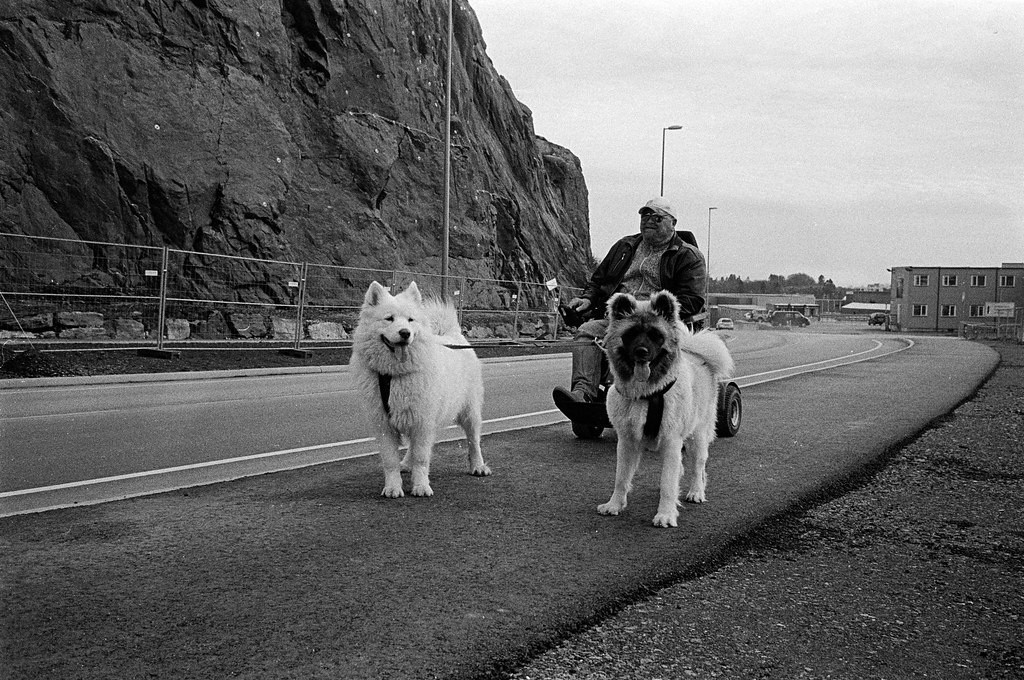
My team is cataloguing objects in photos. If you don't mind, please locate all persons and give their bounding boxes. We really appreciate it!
[552,195,706,404]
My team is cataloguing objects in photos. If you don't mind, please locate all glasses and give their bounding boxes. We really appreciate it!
[639,212,672,224]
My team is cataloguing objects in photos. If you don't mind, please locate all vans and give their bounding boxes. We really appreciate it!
[745,305,811,328]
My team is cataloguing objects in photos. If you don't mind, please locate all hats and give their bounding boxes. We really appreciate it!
[639,197,677,222]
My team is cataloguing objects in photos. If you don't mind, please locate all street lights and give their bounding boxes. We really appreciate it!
[705,206,718,306]
[659,125,683,196]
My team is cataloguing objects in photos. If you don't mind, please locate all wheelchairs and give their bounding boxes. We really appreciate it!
[555,229,742,439]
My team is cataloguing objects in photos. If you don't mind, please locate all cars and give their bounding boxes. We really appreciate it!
[867,313,886,326]
[715,317,734,330]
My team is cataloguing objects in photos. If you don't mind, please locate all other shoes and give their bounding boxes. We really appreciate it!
[552,386,590,402]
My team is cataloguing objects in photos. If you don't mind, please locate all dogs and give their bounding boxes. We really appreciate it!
[349,281,492,500]
[597,289,734,527]
